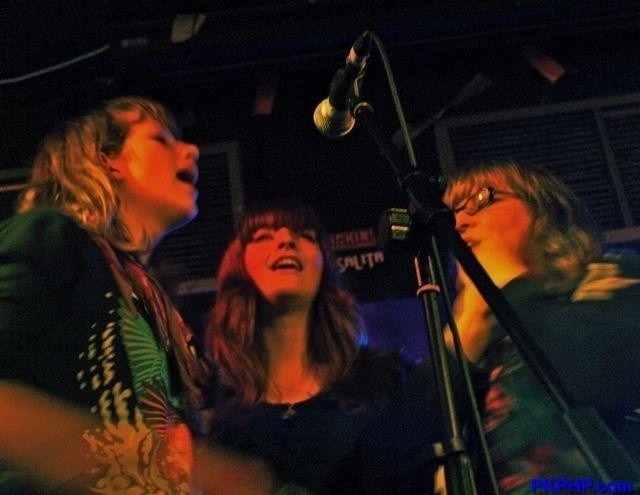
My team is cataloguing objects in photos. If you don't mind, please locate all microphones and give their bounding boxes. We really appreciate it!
[309,38,372,139]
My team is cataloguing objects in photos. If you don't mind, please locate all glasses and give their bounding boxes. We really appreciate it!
[457,187,519,215]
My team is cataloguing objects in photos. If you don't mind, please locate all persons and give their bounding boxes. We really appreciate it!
[441,160,640,493]
[190,192,443,494]
[3,96,276,495]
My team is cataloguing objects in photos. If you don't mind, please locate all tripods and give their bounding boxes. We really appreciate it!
[379,166,640,495]
